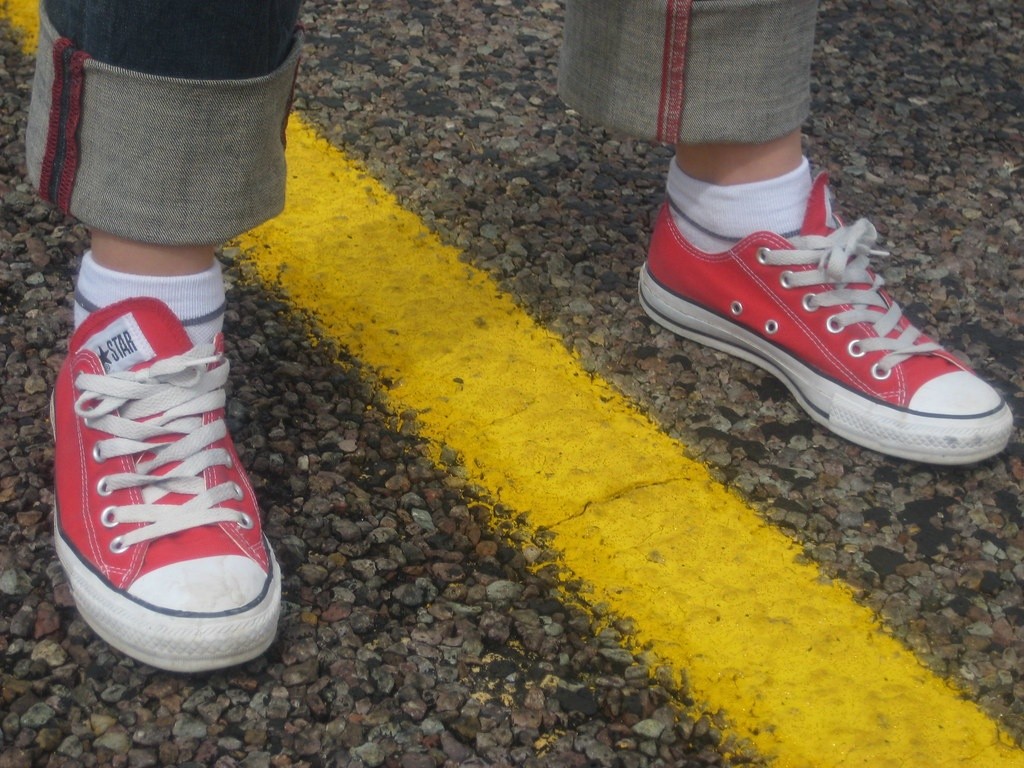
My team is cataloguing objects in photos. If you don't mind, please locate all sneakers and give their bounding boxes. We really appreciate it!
[51,296,281,674]
[639,170,1013,465]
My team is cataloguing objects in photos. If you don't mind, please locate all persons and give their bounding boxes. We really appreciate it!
[23,0,1013,674]
